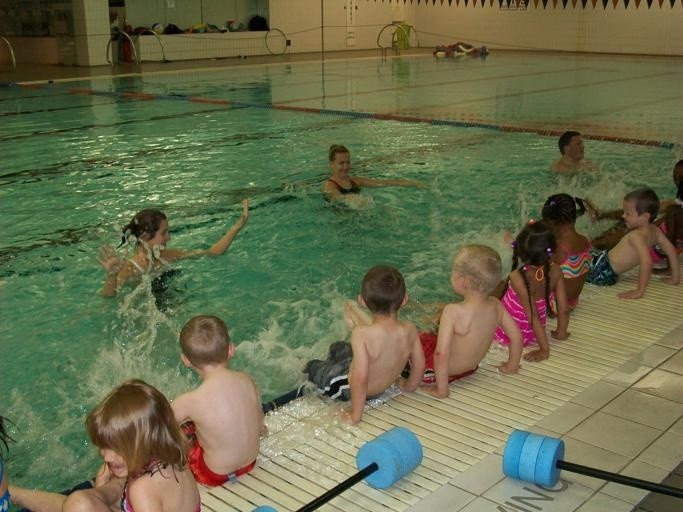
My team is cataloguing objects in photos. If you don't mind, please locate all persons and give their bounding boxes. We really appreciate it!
[167,314,269,488]
[494,219,571,364]
[541,191,591,319]
[546,130,589,185]
[581,158,682,276]
[320,144,424,211]
[303,264,426,425]
[5,377,201,511]
[586,186,680,300]
[340,243,523,399]
[93,195,248,294]
